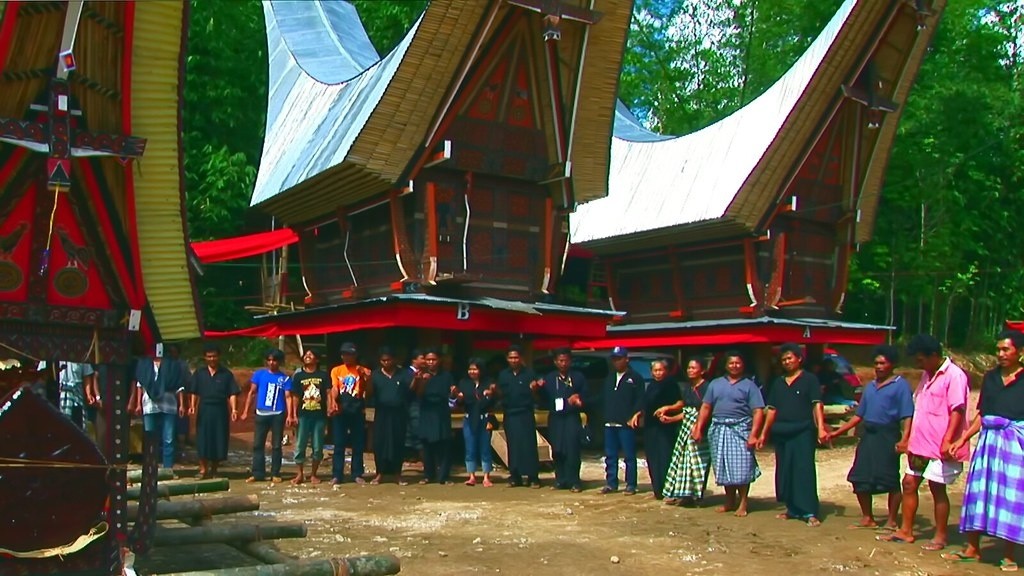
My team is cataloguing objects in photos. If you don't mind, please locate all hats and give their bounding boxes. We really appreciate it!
[339,342,358,355]
[608,347,628,357]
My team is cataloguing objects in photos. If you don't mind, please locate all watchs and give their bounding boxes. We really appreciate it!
[95,395,102,401]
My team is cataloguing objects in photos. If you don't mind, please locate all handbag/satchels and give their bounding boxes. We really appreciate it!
[335,391,367,418]
[481,414,499,431]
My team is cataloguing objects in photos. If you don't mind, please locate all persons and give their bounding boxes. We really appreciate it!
[456,356,502,488]
[536,347,592,494]
[489,343,543,488]
[754,344,829,528]
[950,329,1024,572]
[822,346,915,535]
[239,348,293,485]
[186,341,240,480]
[659,355,712,508]
[689,350,765,518]
[626,356,686,501]
[817,357,859,406]
[875,333,971,550]
[363,345,423,486]
[57,361,108,446]
[127,341,194,480]
[574,347,648,497]
[327,342,374,487]
[402,345,456,486]
[290,347,334,486]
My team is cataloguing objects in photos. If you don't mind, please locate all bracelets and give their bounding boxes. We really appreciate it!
[232,410,238,413]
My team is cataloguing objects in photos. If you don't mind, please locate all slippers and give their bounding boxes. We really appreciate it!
[940,551,978,562]
[194,473,206,481]
[847,521,880,529]
[1000,559,1018,571]
[875,533,914,543]
[596,487,620,494]
[622,490,636,495]
[920,542,950,551]
[875,524,900,534]
